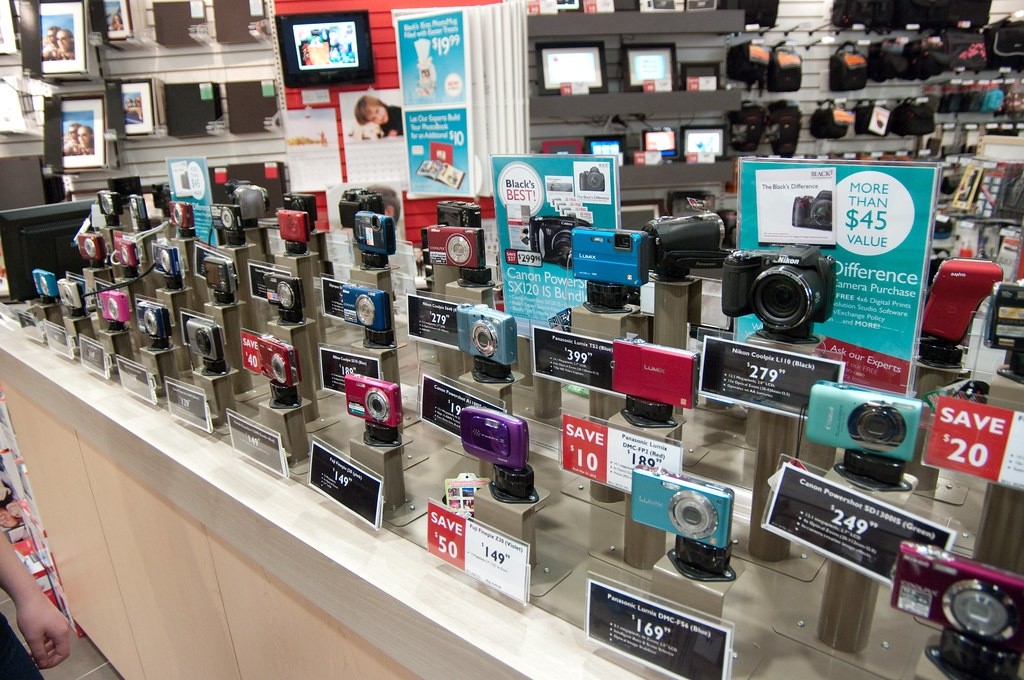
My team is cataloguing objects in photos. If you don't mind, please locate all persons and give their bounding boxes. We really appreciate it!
[124,96,143,123]
[0,530,70,680]
[108,14,124,31]
[354,95,403,138]
[41,26,75,61]
[61,123,94,157]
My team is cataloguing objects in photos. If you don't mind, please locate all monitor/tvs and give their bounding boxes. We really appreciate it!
[0,199,100,302]
[275,10,375,87]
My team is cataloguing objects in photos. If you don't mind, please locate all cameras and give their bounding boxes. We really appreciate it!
[114,239,140,267]
[642,212,729,282]
[204,257,239,293]
[224,177,270,219]
[257,332,302,387]
[96,190,124,215]
[437,200,482,228]
[792,191,832,231]
[457,302,518,365]
[344,372,403,427]
[338,188,385,228]
[572,225,649,286]
[151,183,172,218]
[98,289,131,322]
[341,283,392,331]
[529,216,591,269]
[632,465,734,548]
[154,244,180,274]
[888,540,1024,648]
[283,193,317,221]
[611,336,701,409]
[922,259,1003,344]
[32,268,60,297]
[77,233,107,260]
[420,225,486,269]
[57,278,86,308]
[127,194,148,219]
[278,210,311,243]
[984,281,1024,349]
[136,302,173,338]
[805,379,923,463]
[168,201,194,227]
[460,405,529,470]
[579,167,605,191]
[721,245,837,331]
[353,210,396,255]
[263,272,306,311]
[186,318,226,361]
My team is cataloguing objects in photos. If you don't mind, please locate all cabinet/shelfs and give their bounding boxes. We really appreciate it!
[0,269,1024,680]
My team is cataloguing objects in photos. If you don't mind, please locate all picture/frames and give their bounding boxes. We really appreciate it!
[37,0,93,78]
[620,199,666,232]
[541,139,583,154]
[53,92,108,173]
[102,0,135,41]
[120,98,166,137]
[622,42,679,93]
[585,134,630,166]
[642,127,679,157]
[680,125,728,163]
[681,62,721,92]
[534,40,610,96]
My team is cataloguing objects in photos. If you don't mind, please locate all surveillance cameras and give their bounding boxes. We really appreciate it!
[209,204,244,230]
